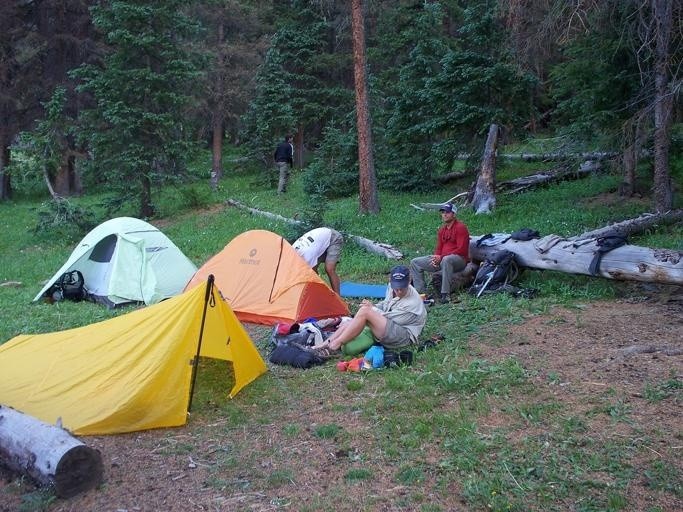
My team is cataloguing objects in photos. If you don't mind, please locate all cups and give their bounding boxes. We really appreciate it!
[400,350,413,366]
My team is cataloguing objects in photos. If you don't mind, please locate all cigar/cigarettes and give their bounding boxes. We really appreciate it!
[432,261,436,265]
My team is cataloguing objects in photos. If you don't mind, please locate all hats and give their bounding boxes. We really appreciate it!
[390,265,410,289]
[439,203,458,215]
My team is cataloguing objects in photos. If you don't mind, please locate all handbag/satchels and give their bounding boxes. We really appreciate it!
[588,232,629,278]
[511,228,540,240]
[270,343,323,369]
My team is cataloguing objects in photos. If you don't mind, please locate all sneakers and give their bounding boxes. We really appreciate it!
[313,344,340,358]
[441,293,452,304]
[294,342,312,353]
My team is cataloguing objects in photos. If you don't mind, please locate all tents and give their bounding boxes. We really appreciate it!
[0,274,269,432]
[181,229,350,324]
[33,217,197,306]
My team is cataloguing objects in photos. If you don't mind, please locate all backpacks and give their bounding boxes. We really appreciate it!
[44,270,96,304]
[476,249,519,294]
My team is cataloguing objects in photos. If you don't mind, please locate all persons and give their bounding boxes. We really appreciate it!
[274,134,295,192]
[293,227,344,296]
[410,203,470,305]
[307,266,428,357]
